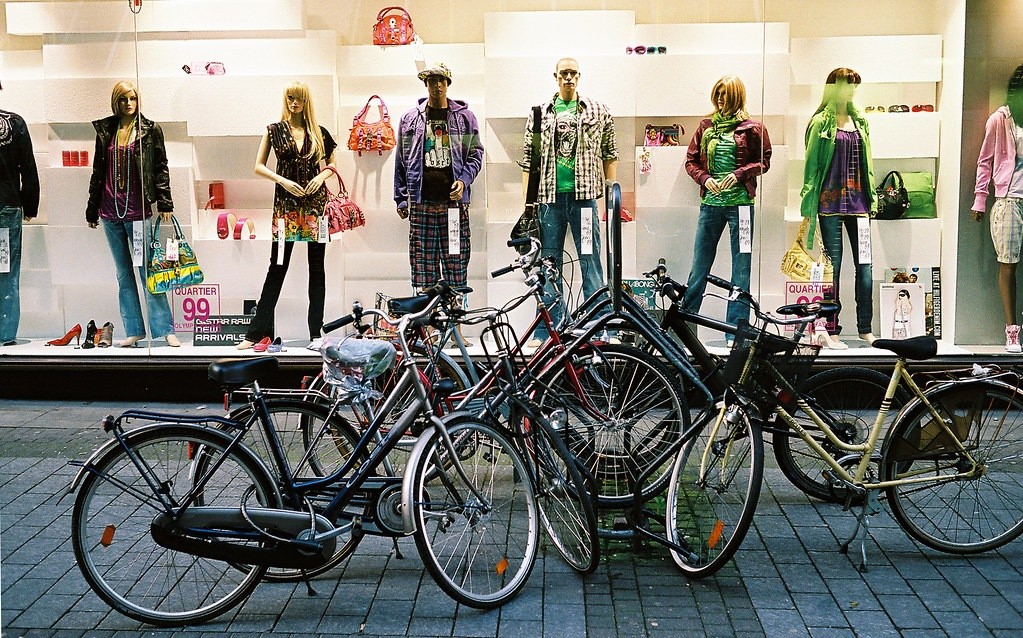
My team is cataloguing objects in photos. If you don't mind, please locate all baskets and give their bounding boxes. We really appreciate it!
[725,321,821,412]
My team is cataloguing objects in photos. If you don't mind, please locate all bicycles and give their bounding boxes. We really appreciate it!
[57,238,1022,627]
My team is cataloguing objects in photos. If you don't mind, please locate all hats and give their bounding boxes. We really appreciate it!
[418,62,452,86]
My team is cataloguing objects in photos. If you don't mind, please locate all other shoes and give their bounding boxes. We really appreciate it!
[254,337,271,352]
[267,337,284,352]
[98,322,114,347]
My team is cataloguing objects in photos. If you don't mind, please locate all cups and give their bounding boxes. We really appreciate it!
[70,151,79,166]
[62,150,71,166]
[79,150,89,167]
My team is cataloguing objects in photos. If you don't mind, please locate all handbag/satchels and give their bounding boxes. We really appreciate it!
[779,223,829,287]
[321,167,366,234]
[372,6,419,45]
[643,123,684,146]
[509,204,540,255]
[872,171,910,220]
[147,212,204,294]
[348,94,396,152]
[181,59,226,75]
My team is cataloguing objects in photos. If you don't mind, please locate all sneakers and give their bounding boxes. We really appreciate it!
[1003,325,1022,353]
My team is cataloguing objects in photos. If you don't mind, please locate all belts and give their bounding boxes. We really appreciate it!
[217,213,257,239]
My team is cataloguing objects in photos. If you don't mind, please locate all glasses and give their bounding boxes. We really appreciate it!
[865,103,936,114]
[624,45,667,54]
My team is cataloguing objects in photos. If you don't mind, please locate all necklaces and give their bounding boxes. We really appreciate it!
[115,115,138,218]
[287,119,308,202]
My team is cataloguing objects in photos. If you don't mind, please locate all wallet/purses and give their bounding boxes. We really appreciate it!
[209,183,226,209]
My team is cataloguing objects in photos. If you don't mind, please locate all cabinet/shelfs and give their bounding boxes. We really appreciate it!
[0,2,966,357]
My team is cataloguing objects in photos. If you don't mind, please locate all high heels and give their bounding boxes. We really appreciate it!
[82,320,98,349]
[47,324,82,347]
[813,324,847,349]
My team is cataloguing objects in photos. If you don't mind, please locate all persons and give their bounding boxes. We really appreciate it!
[393,65,483,347]
[85,82,181,348]
[800,67,878,350]
[971,66,1023,353]
[681,75,772,348]
[517,57,623,345]
[0,83,39,346]
[234,84,337,351]
